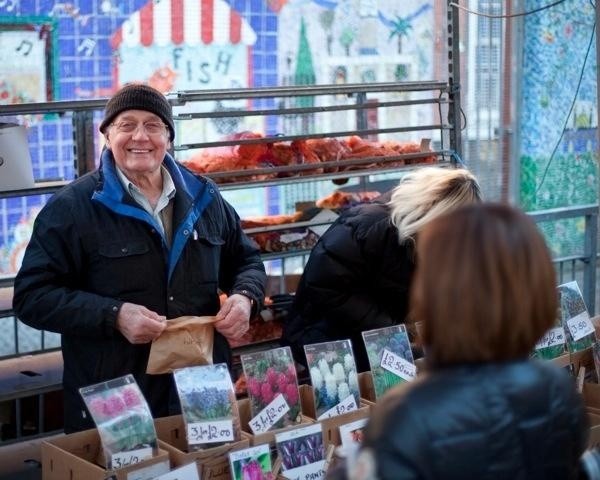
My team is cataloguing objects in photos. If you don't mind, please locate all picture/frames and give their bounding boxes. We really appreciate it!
[0,14,59,124]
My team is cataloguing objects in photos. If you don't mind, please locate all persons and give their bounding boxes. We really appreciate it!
[12,84,267,434]
[279,166,483,373]
[354,202,591,480]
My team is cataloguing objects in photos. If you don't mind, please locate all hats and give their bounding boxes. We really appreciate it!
[99,85,175,142]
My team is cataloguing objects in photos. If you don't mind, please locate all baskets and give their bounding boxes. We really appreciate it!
[255,233,317,251]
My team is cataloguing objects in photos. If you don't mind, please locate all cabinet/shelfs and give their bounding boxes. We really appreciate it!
[0,0,463,447]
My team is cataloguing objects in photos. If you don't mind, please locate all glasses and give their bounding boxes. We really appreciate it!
[111,121,169,132]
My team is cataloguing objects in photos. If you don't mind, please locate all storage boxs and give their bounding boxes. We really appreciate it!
[42,315,599,480]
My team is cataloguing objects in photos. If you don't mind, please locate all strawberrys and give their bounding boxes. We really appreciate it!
[249,362,298,408]
